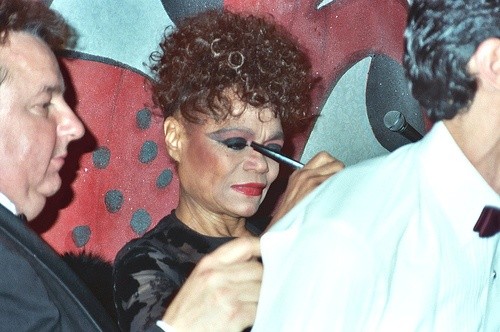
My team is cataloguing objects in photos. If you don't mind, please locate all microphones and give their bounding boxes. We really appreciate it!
[384,110,423,142]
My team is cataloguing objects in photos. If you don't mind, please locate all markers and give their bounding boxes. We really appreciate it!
[249,140,306,172]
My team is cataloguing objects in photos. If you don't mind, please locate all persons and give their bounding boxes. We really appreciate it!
[0,0,263,332]
[113,8,346,332]
[253,1,499,331]
